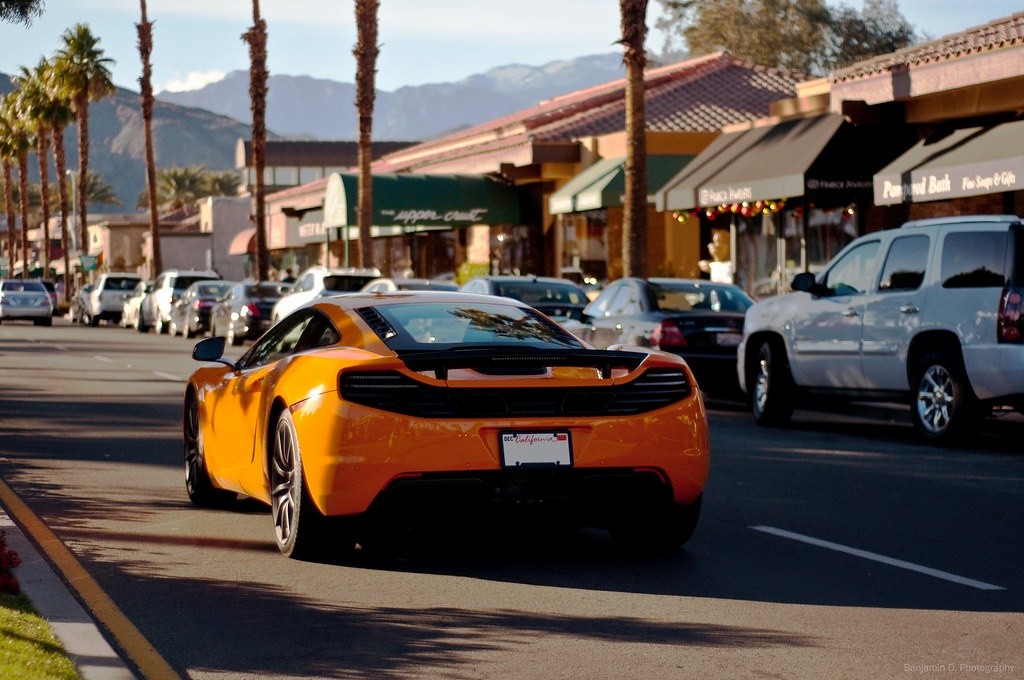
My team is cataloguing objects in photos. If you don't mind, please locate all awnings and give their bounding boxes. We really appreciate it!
[228,228,257,256]
[873,120,1024,207]
[655,113,873,211]
[548,156,700,216]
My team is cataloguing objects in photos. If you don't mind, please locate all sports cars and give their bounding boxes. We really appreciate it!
[183,291,710,560]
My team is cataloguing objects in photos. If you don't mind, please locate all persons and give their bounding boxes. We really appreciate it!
[280,268,296,292]
[269,270,278,281]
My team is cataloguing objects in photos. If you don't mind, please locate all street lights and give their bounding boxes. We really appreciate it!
[66,169,77,252]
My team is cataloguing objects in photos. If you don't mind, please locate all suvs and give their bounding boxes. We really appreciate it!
[136,268,220,334]
[736,215,1023,437]
[42,281,58,315]
[268,264,382,352]
[86,274,142,326]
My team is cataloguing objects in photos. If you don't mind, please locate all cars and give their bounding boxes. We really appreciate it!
[555,276,757,396]
[69,282,93,325]
[0,280,52,326]
[209,281,293,346]
[120,280,155,329]
[169,282,228,338]
[459,276,590,323]
[360,277,459,293]
